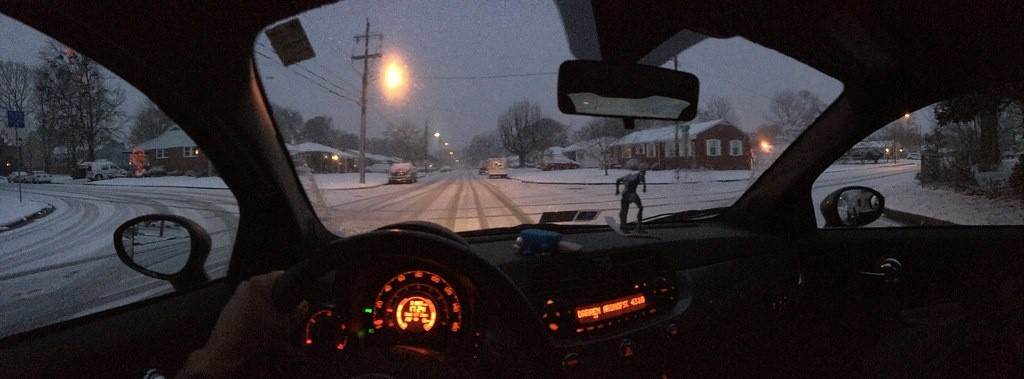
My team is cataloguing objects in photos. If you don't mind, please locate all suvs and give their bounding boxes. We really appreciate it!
[388,163,418,184]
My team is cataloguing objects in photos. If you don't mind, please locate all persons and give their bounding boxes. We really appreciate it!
[144,270,309,378]
[615,171,647,234]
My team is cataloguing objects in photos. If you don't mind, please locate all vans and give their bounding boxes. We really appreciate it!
[86,161,121,181]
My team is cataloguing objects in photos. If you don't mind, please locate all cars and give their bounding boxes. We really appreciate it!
[907,153,922,160]
[22,170,53,184]
[479,165,488,174]
[540,157,579,171]
[6,171,29,184]
[511,162,529,168]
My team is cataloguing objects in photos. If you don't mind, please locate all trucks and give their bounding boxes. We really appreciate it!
[487,157,508,178]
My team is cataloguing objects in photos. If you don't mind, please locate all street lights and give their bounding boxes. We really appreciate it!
[904,114,910,153]
[360,63,405,184]
